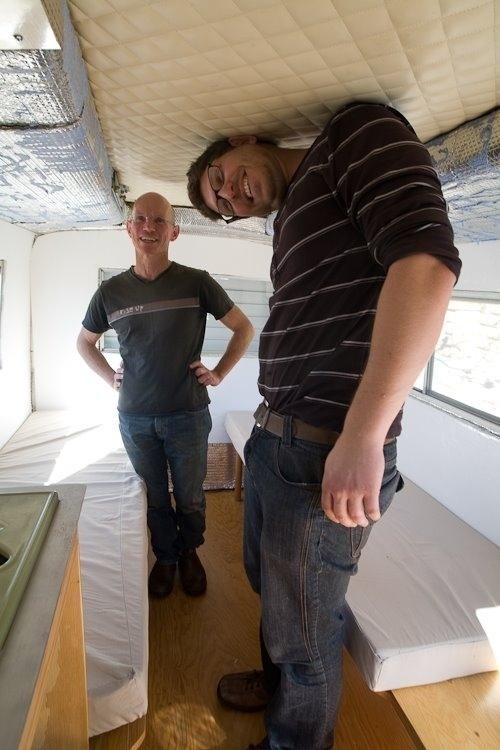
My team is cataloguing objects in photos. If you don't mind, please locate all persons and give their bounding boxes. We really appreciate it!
[73,192,256,598]
[184,98,462,750]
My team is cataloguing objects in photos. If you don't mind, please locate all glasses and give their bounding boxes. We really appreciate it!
[206,155,239,225]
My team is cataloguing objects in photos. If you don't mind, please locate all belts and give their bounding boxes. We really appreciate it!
[252,398,394,446]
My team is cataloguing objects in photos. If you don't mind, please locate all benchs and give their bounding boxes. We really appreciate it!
[1,403,149,749]
[221,393,500,692]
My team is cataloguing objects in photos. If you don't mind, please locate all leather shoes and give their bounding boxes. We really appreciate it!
[217,670,266,713]
[177,548,207,598]
[147,558,176,601]
